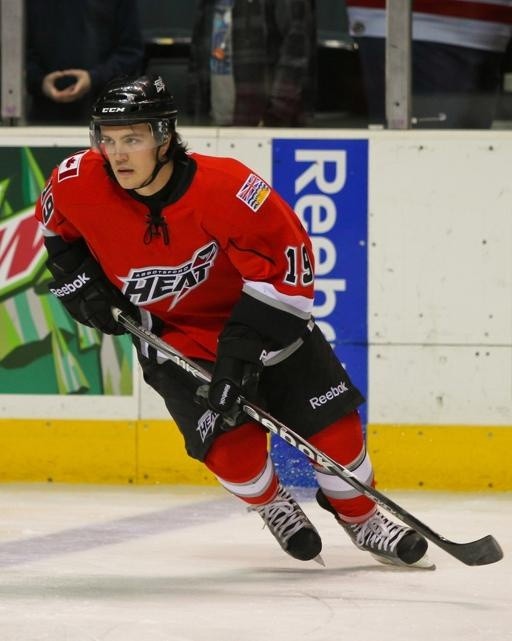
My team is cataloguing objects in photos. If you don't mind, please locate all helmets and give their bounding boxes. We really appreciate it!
[88,71,178,164]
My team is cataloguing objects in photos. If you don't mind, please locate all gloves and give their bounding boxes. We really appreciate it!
[192,324,266,431]
[45,235,142,336]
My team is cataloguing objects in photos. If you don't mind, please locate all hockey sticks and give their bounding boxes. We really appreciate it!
[112,309,503,567]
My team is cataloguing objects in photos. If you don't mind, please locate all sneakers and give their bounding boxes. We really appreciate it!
[315,486,428,565]
[246,484,322,560]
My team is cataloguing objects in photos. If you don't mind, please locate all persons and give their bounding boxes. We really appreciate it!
[33,64,436,565]
[191,0,317,127]
[25,0,160,127]
